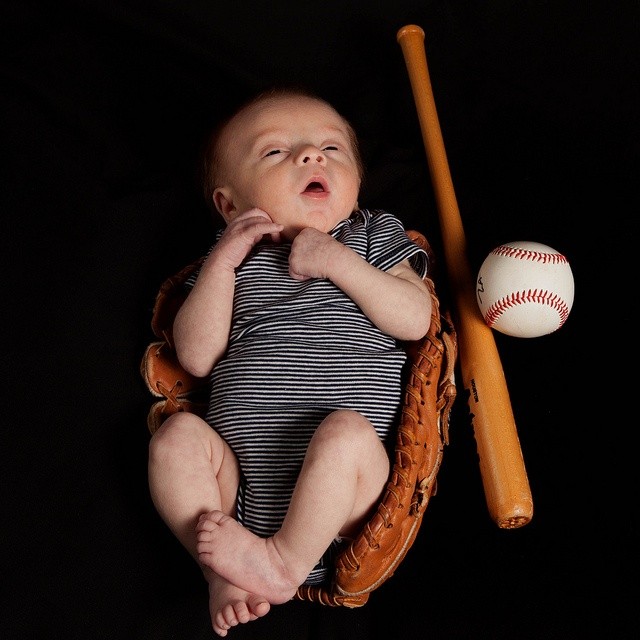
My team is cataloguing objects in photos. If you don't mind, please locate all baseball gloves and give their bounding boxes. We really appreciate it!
[135,231,460,607]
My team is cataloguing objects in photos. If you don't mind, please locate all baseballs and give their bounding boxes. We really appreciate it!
[476,241,578,339]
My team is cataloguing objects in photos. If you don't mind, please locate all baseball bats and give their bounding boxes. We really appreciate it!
[392,23,536,530]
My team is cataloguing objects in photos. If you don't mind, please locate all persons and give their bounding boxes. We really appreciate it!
[148,89,432,636]
